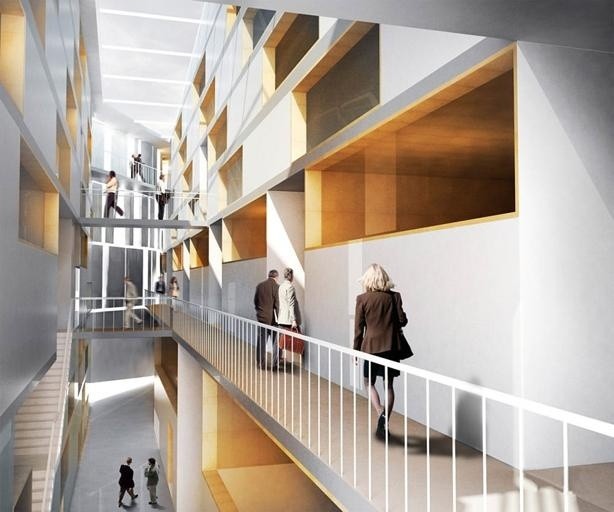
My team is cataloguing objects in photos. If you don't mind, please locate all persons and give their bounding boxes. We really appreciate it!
[353,263,414,438]
[134,153,146,182]
[155,175,171,220]
[130,154,136,180]
[117,457,138,508]
[143,457,160,504]
[122,276,143,330]
[253,269,279,371]
[154,276,165,306]
[271,268,304,372]
[104,171,125,219]
[167,276,179,312]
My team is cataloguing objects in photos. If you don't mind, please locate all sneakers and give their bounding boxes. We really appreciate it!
[149,496,158,504]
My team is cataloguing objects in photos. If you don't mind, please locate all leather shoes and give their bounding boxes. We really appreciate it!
[376,410,390,438]
[132,495,138,500]
[119,502,123,506]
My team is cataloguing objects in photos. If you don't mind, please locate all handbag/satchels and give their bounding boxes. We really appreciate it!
[280,328,304,353]
[396,331,414,360]
